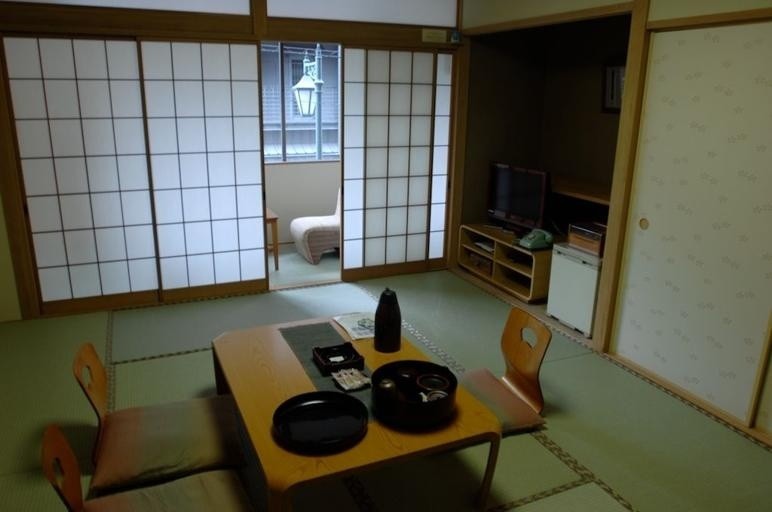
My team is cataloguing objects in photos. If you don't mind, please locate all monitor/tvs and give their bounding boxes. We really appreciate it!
[487,162,547,233]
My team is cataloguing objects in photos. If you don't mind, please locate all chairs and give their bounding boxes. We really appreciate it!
[35,342,252,509]
[460,306,552,439]
[289,185,341,266]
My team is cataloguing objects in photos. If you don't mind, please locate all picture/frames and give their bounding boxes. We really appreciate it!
[599,61,626,115]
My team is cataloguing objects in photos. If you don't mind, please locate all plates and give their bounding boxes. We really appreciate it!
[271,390,369,456]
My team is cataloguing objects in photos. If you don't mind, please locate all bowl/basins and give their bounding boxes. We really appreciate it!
[370,359,458,427]
[416,374,450,391]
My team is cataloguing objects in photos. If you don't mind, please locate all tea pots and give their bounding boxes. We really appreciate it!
[418,390,449,403]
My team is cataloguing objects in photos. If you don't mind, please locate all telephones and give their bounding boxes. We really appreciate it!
[519,229,554,250]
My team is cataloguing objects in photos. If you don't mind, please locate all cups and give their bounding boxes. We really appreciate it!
[378,378,397,399]
[396,366,417,393]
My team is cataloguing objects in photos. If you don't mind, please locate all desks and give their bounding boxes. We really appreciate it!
[211,310,501,512]
[543,242,601,337]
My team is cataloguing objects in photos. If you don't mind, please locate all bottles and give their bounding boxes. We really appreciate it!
[373,286,402,354]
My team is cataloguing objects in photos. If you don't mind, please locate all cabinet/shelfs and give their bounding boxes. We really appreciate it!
[455,223,551,303]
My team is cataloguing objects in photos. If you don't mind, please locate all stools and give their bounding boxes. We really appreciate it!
[266,208,279,270]
